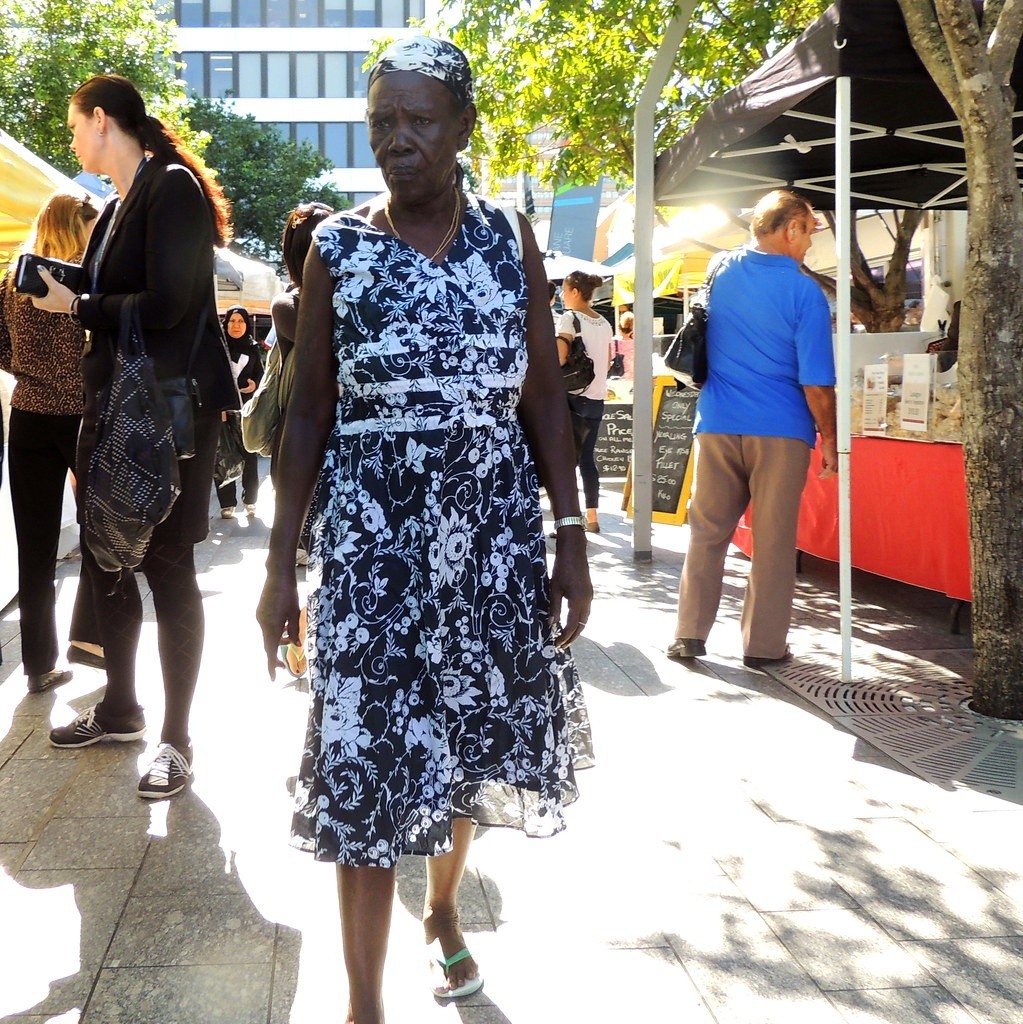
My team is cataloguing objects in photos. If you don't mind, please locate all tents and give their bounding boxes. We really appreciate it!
[0,126,292,314]
[654,0,1023,683]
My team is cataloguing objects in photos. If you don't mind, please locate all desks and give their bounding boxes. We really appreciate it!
[797,432,973,634]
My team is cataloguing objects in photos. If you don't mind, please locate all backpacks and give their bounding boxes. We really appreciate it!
[240,341,294,458]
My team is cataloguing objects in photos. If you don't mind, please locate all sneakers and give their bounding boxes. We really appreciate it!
[137,737,194,799]
[48,702,147,747]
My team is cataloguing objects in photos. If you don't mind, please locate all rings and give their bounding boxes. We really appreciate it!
[578,621,587,627]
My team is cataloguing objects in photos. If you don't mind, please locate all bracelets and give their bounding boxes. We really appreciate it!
[69,295,82,326]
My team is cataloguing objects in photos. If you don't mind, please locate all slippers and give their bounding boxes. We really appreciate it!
[424,947,483,998]
[281,608,307,678]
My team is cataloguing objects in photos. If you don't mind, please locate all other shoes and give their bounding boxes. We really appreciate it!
[585,522,600,532]
[243,502,256,517]
[667,638,706,656]
[28,669,73,695]
[66,637,107,669]
[220,507,235,519]
[744,643,791,667]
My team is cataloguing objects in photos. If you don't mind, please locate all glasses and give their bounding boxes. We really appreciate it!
[294,202,334,218]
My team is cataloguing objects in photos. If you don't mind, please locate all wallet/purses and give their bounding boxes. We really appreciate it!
[13,253,84,298]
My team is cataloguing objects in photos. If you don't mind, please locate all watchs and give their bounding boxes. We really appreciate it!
[555,515,589,534]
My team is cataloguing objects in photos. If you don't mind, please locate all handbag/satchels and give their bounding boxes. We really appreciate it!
[664,250,731,391]
[214,409,245,490]
[84,294,207,567]
[560,311,595,398]
[607,340,625,380]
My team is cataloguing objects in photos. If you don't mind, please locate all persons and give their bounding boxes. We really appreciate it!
[667,190,838,668]
[0,195,107,692]
[257,35,594,1024]
[535,269,634,534]
[928,301,960,372]
[215,204,337,521]
[31,73,241,799]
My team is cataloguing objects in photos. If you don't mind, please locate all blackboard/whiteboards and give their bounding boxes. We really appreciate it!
[592,403,634,478]
[626,377,699,526]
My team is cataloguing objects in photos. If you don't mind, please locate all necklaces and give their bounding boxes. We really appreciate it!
[384,188,461,267]
